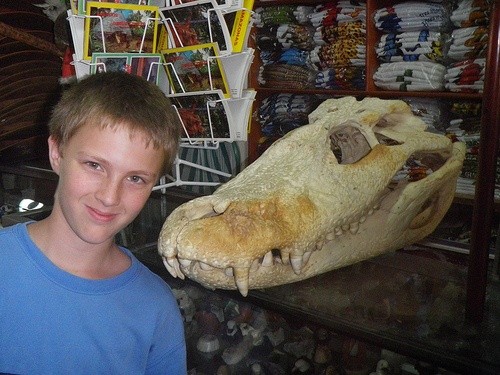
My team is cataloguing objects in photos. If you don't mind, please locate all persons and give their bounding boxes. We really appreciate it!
[0,70,186,375]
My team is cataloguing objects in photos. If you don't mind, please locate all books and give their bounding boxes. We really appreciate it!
[67,0,257,143]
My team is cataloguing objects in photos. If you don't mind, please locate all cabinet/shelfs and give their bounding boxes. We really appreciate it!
[0,0,500,375]
